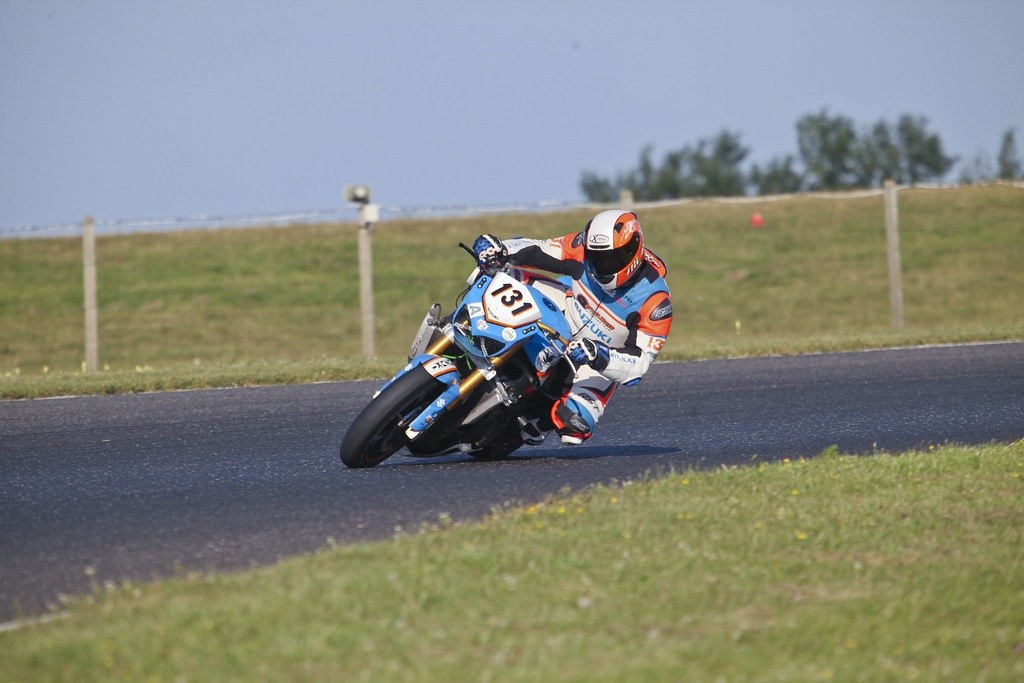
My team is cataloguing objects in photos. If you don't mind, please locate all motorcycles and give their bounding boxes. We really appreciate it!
[339,241,590,469]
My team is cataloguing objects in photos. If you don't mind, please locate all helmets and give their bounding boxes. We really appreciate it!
[584,210,644,290]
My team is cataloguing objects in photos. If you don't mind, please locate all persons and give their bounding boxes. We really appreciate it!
[473,209,674,444]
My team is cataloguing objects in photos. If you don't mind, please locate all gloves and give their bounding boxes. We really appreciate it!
[473,234,508,264]
[566,337,609,370]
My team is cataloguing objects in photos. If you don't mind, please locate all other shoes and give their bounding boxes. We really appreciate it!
[521,418,553,445]
